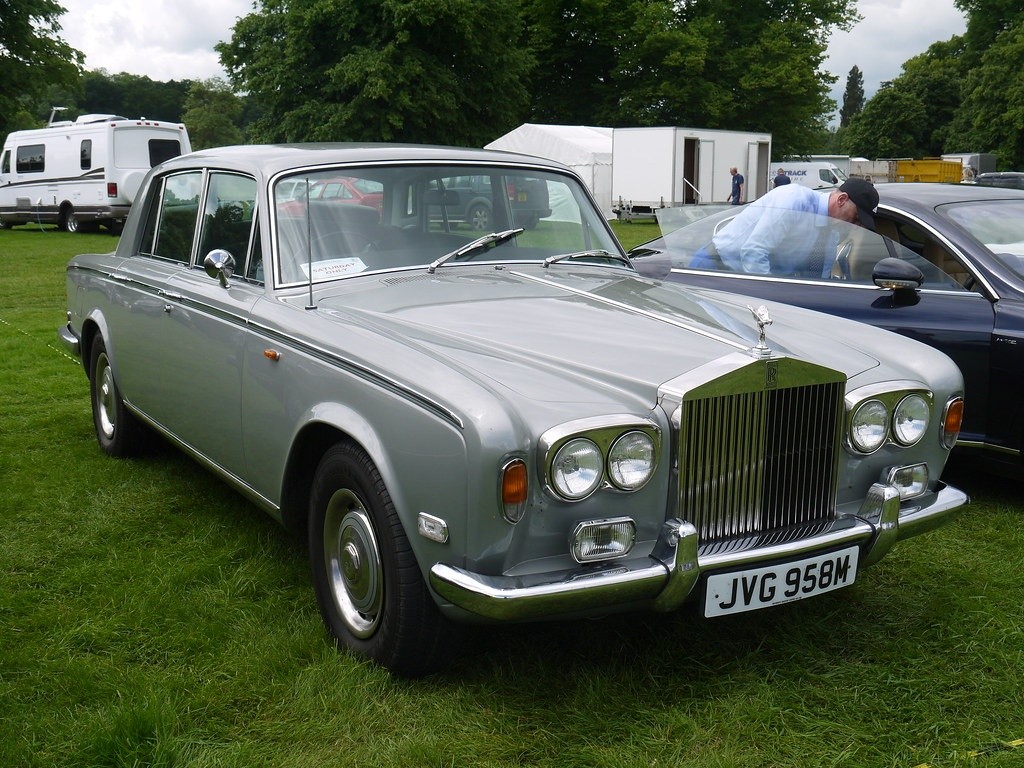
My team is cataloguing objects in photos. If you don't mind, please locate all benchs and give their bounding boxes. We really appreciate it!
[154,203,413,276]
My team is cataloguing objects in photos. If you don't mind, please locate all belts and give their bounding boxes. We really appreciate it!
[706,241,724,270]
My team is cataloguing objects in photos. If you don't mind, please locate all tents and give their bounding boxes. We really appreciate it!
[483,124,613,223]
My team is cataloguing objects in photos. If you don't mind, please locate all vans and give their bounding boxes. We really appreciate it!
[770,162,848,191]
[0,106,193,232]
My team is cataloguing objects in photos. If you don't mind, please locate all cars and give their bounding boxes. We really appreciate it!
[276,179,314,204]
[626,183,1024,469]
[277,177,384,216]
[60,142,969,660]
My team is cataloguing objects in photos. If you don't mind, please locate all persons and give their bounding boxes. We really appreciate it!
[688,178,879,279]
[960,168,978,184]
[774,168,791,187]
[730,168,744,205]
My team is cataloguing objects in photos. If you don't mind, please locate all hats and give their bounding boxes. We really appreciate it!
[839,177,880,231]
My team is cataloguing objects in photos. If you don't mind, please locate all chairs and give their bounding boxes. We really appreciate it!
[923,236,977,292]
[830,218,903,281]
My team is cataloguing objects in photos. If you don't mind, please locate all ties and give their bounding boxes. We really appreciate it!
[807,226,833,278]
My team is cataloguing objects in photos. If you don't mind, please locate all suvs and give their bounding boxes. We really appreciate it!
[428,174,552,231]
